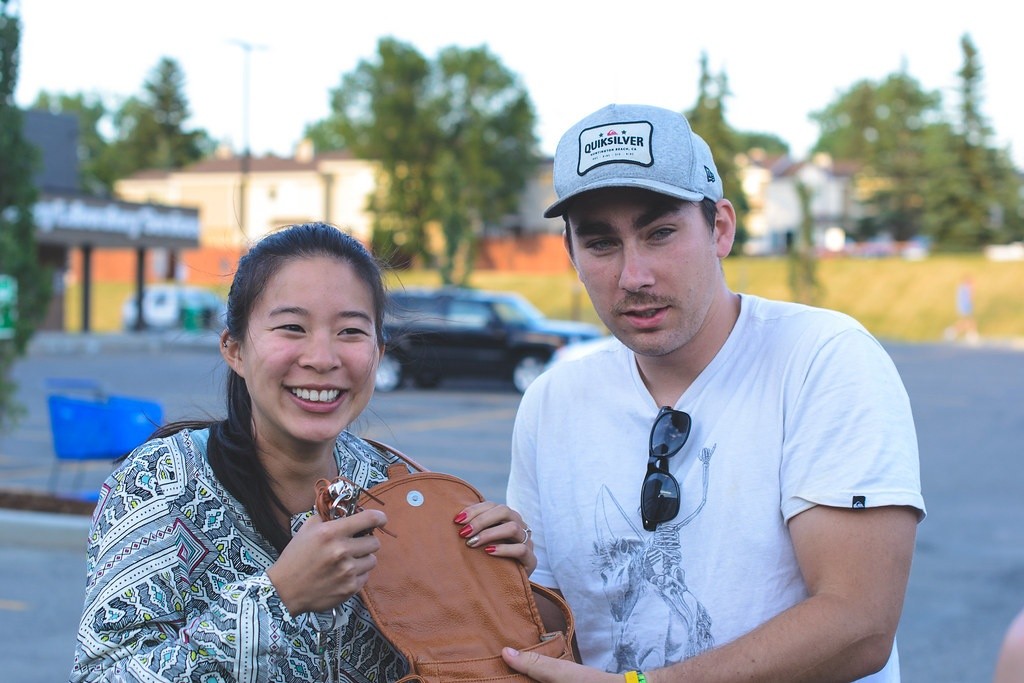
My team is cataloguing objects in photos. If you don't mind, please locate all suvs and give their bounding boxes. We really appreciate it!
[372,290,599,392]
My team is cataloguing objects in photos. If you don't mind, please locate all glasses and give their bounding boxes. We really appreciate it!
[641,405,691,531]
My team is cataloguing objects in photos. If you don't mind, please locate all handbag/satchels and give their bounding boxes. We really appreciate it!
[343,434,586,683]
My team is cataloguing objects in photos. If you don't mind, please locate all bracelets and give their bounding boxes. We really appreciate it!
[625,670,647,683]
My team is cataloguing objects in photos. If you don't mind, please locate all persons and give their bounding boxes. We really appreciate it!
[506,105,929,683]
[69,221,537,683]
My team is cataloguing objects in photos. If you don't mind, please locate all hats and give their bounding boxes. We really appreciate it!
[543,105,724,219]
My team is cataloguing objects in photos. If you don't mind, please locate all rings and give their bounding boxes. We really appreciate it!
[522,527,532,544]
